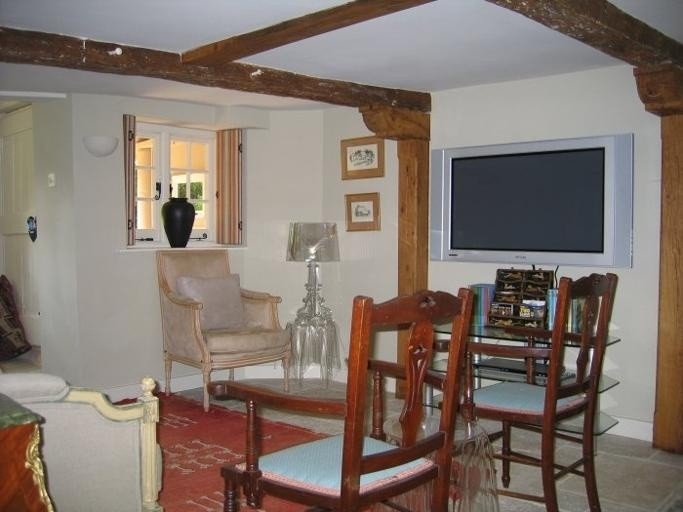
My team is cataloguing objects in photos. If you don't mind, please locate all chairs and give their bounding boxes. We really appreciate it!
[154,249,292,413]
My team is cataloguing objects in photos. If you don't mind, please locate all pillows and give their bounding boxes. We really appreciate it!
[174,274,245,331]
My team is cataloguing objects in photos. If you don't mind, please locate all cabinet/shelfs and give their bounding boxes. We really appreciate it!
[424,329,621,458]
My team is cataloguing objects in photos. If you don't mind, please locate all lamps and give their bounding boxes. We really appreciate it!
[81,134,119,157]
[282,220,342,388]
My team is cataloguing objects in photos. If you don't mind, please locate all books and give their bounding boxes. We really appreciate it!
[466,269,604,338]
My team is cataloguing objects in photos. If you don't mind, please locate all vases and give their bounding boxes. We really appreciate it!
[160,197,196,249]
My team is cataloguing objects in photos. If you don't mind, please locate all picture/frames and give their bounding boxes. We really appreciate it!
[339,137,384,181]
[343,192,381,232]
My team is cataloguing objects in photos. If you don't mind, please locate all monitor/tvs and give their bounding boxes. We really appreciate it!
[430,134,633,269]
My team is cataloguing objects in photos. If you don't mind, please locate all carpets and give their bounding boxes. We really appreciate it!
[110,391,498,511]
